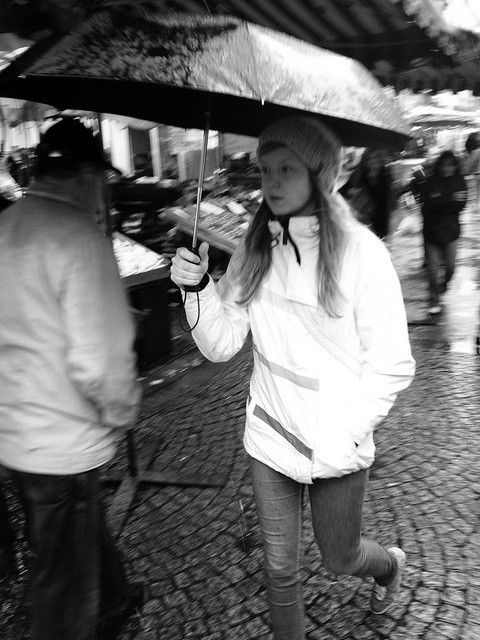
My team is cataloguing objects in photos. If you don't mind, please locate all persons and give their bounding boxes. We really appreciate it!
[1,115,151,637]
[409,150,469,316]
[337,143,393,246]
[169,118,418,640]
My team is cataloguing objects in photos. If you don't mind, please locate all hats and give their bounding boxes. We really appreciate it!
[34,119,121,177]
[256,116,342,196]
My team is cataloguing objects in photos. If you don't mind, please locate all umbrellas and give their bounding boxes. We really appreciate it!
[2,5,429,333]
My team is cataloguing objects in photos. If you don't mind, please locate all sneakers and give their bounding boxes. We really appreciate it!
[96,581,145,640]
[370,547,406,615]
[427,304,442,312]
